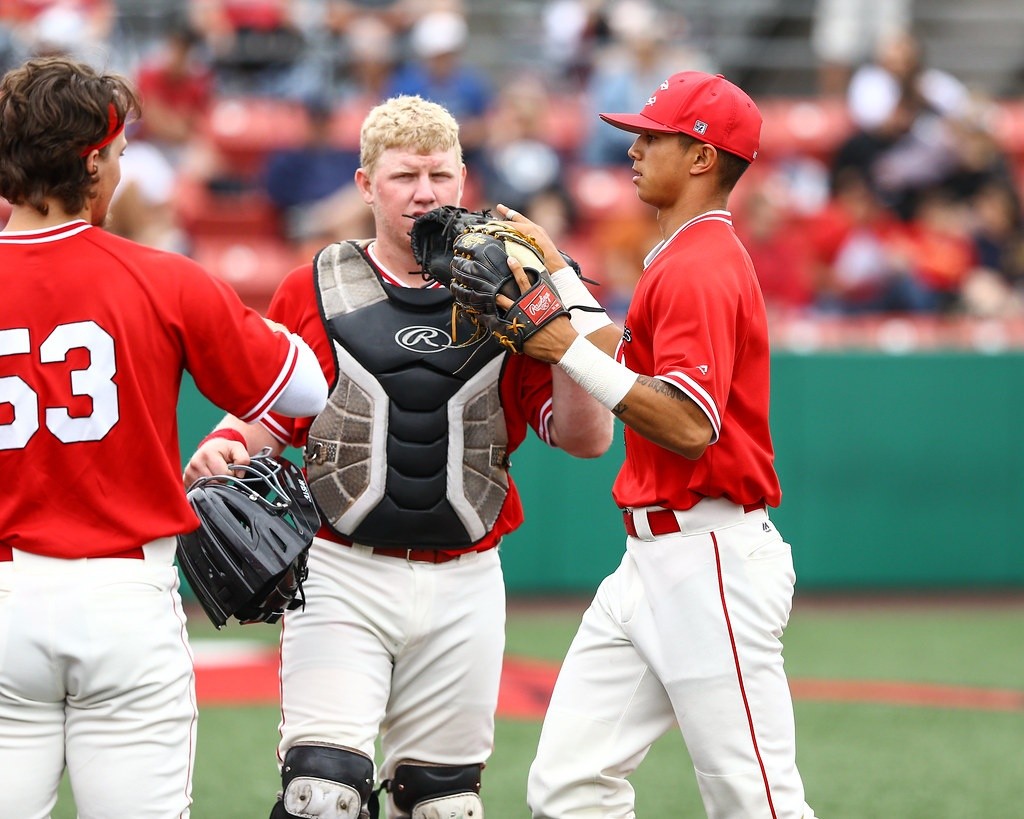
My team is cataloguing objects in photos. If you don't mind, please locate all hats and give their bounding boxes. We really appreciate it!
[599,71,762,164]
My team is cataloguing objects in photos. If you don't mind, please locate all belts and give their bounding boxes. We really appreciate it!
[0,539,145,562]
[619,492,768,539]
[316,523,458,569]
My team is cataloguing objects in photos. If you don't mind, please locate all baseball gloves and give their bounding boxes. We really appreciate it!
[408,207,585,349]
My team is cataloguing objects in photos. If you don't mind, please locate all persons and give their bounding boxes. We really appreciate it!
[454,70,816,819]
[184,94,614,819]
[0,61,328,819]
[0,0,1024,338]
